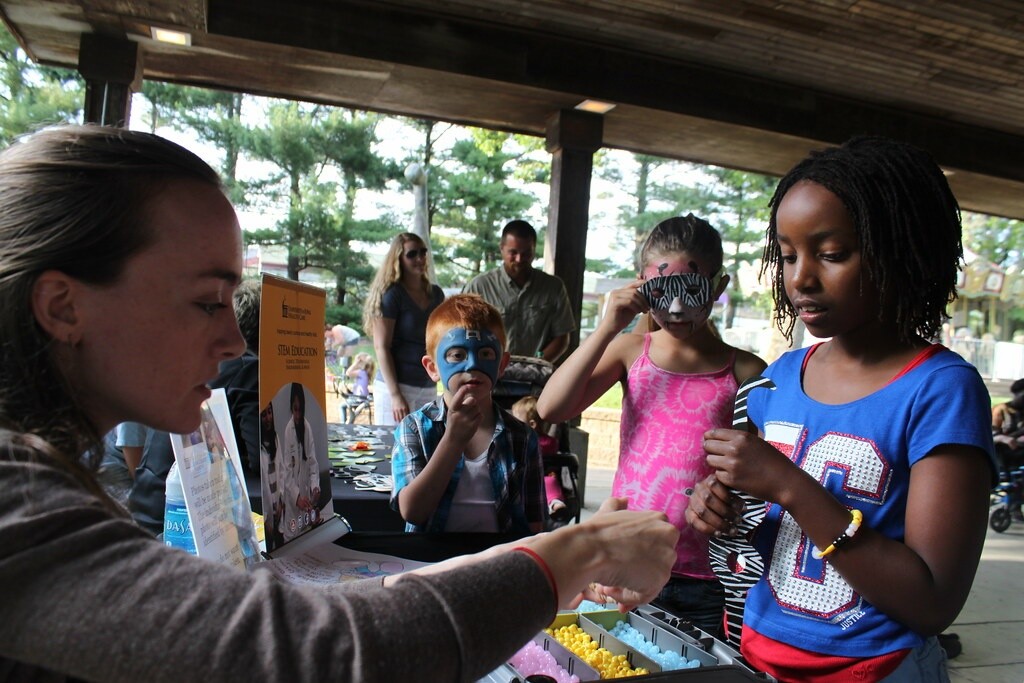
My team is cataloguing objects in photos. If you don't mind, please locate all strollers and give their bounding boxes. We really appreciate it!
[490,354,583,533]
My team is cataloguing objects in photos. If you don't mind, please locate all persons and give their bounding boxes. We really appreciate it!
[207,272,260,479]
[338,354,375,424]
[0,122,680,683]
[536,212,769,643]
[326,318,360,356]
[683,133,997,683]
[462,219,577,363]
[392,294,546,533]
[992,378,1024,522]
[82,422,146,508]
[362,231,447,426]
[509,394,567,515]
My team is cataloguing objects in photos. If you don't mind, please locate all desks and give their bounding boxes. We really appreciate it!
[246,423,408,534]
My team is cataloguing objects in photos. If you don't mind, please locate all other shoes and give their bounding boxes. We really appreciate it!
[548,500,566,515]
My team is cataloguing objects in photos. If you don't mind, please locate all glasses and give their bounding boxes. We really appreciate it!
[404,248,428,258]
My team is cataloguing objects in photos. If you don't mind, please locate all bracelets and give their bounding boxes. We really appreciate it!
[512,547,560,629]
[811,509,863,559]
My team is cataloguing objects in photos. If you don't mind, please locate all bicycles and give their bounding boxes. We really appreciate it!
[989,457,1024,536]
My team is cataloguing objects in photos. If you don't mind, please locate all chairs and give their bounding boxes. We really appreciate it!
[332,376,373,425]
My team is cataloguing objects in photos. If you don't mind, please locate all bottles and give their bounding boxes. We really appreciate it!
[162,461,197,558]
[534,352,545,369]
